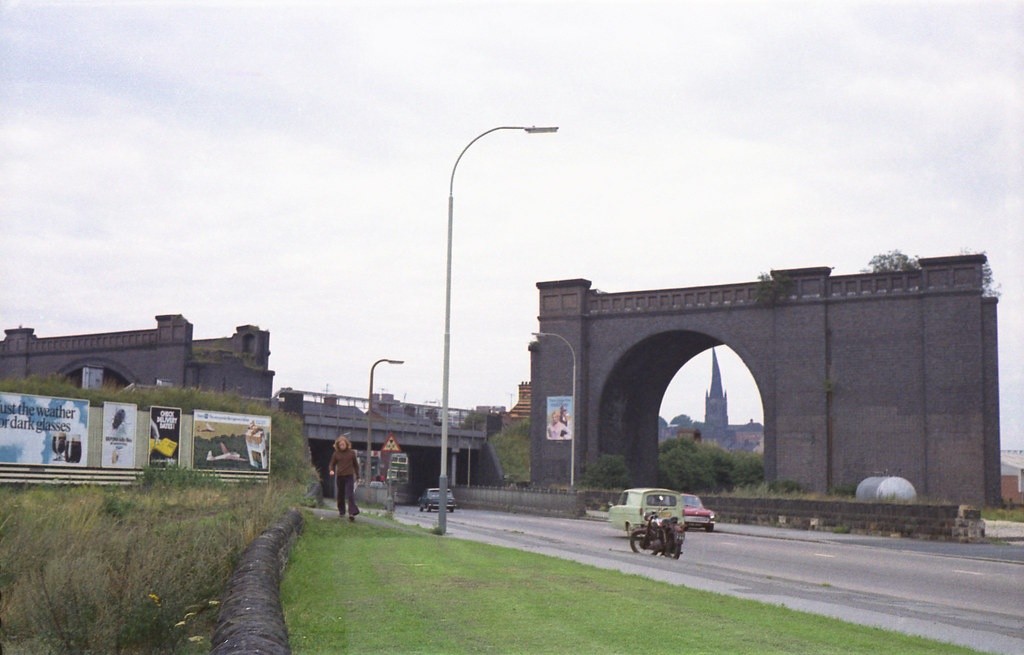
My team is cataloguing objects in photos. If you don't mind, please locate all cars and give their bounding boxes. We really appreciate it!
[681,493,716,531]
[609,487,682,533]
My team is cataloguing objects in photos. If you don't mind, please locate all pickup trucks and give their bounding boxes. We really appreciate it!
[418,489,457,513]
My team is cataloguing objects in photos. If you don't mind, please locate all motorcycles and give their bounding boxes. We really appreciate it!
[630,508,684,559]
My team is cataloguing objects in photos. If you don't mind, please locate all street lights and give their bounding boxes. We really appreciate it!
[438,125,559,534]
[364,358,404,487]
[532,331,576,485]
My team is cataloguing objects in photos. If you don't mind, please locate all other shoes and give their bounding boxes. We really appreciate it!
[349,515,354,522]
[339,514,343,518]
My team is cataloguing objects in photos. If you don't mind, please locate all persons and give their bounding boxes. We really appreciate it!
[329,437,361,521]
[547,405,572,439]
[112,409,133,465]
